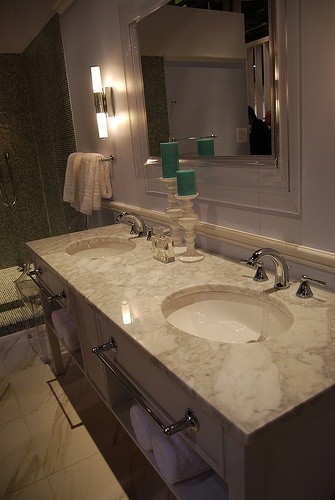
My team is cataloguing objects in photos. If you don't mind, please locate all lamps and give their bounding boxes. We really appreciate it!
[91,66,114,140]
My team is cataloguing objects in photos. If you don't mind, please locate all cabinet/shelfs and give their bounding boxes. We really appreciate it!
[26,255,335,500]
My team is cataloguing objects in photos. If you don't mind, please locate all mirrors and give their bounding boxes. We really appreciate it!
[129,0,291,168]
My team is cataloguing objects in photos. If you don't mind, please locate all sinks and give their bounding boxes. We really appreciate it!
[63,235,136,261]
[159,282,295,345]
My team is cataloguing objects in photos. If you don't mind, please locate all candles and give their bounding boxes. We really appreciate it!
[196,139,215,156]
[160,141,179,178]
[176,169,197,197]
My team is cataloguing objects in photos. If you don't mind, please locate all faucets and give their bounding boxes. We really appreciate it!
[115,211,148,237]
[246,247,291,289]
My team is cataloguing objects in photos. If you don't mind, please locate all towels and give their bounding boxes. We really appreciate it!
[50,308,79,352]
[129,400,213,486]
[62,151,113,216]
